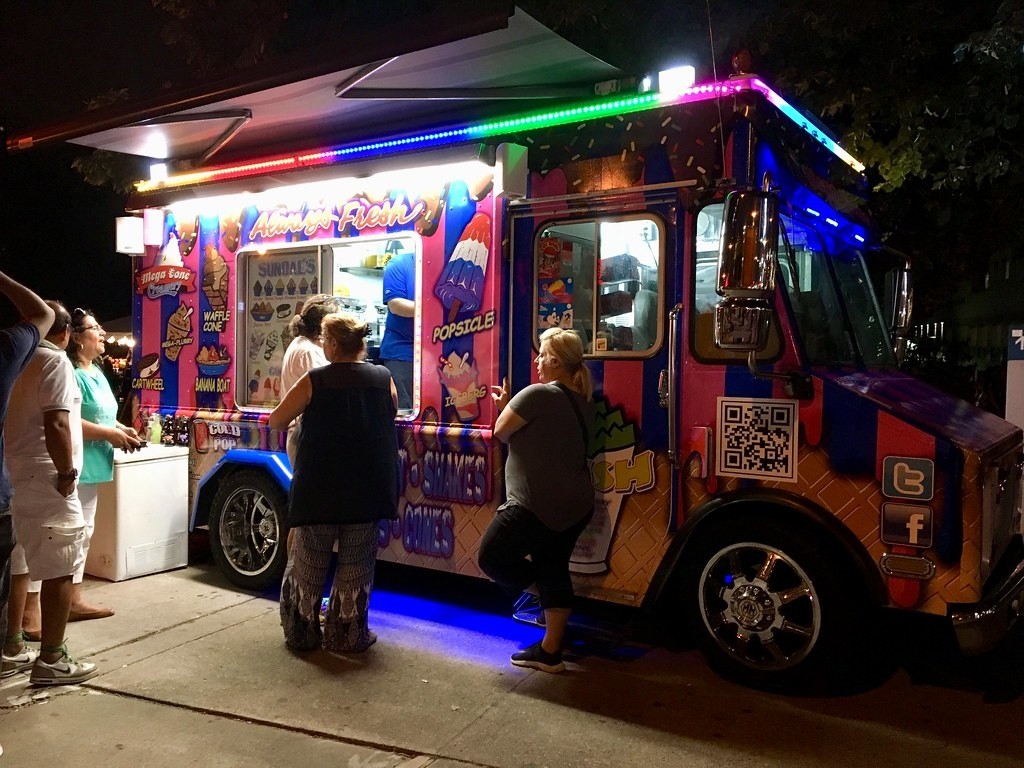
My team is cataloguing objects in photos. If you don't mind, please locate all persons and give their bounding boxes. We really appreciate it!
[266,291,403,657]
[477,327,610,672]
[379,255,416,415]
[0,272,142,688]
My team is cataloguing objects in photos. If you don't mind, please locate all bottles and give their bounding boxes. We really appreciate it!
[248,369,261,402]
[147,413,189,446]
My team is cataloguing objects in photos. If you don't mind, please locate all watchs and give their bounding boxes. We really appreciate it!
[58,468,78,481]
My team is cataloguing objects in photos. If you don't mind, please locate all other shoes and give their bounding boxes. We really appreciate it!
[68,607,115,622]
[23,629,42,641]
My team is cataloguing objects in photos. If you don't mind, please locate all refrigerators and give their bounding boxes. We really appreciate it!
[83,443,189,583]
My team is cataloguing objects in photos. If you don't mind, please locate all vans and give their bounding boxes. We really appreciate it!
[115,46,1024,693]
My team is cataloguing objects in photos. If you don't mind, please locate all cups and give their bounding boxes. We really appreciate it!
[249,333,265,360]
[263,337,277,361]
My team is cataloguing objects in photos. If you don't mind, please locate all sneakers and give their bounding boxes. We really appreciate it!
[510,645,566,673]
[30,649,100,684]
[0,644,42,678]
[535,615,546,626]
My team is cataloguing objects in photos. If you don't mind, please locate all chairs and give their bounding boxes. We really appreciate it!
[632,290,657,351]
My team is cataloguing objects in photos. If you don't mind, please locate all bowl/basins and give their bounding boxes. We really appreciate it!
[250,310,273,321]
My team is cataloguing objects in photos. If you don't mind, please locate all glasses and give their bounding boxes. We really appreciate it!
[318,335,330,342]
[78,325,103,333]
